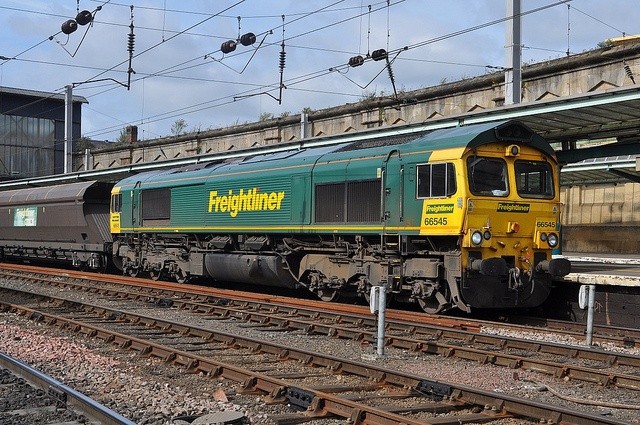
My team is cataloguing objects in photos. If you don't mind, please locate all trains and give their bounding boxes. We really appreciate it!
[0,118,571,315]
